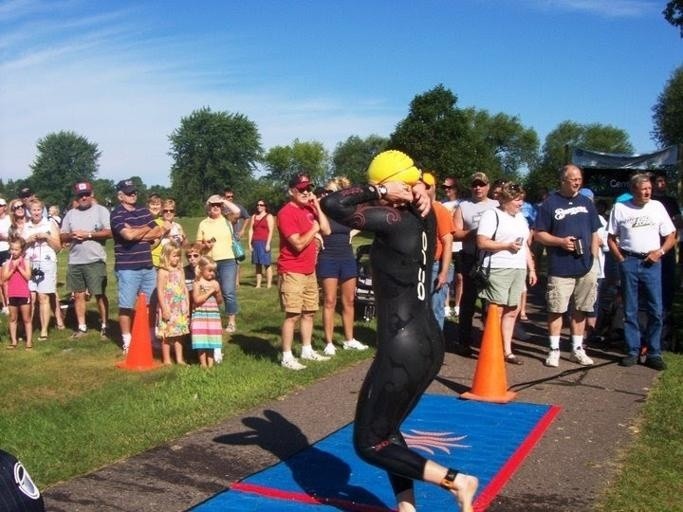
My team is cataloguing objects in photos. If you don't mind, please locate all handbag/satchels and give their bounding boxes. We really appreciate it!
[469,264,491,293]
[232,233,244,261]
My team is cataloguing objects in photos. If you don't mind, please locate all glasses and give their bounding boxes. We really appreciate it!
[163,210,175,213]
[210,203,222,207]
[257,204,265,206]
[441,186,454,189]
[227,196,234,198]
[124,191,137,195]
[298,188,312,192]
[508,185,519,190]
[0,204,7,207]
[14,205,24,209]
[78,192,91,197]
[187,254,199,258]
[471,183,486,188]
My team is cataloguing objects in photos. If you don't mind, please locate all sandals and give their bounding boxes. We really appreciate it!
[504,353,524,365]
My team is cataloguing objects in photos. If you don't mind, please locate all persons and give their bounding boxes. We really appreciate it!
[276,163,683,371]
[110,180,249,369]
[0,181,112,349]
[320,149,479,511]
[249,199,274,288]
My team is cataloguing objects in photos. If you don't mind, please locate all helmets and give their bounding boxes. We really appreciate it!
[422,173,435,185]
[368,150,420,185]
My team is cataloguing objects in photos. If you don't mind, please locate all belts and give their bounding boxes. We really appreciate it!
[618,248,650,259]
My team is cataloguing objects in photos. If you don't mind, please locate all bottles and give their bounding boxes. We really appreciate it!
[231,239,245,261]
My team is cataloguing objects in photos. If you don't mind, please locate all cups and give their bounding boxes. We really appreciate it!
[573,238,584,255]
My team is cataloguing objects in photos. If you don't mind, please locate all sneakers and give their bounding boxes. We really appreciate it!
[123,346,130,355]
[100,328,112,343]
[324,342,337,355]
[546,347,560,367]
[301,346,331,361]
[644,355,668,371]
[570,346,593,365]
[281,352,307,370]
[68,330,88,341]
[619,354,641,366]
[343,338,369,350]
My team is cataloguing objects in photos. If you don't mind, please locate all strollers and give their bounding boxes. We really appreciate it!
[355,246,375,320]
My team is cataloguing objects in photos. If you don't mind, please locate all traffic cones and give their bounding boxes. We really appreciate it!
[114,293,163,372]
[460,305,516,404]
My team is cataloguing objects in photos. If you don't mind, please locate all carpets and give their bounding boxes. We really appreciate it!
[185,395,560,512]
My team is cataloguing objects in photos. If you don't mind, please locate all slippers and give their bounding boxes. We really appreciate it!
[38,336,48,341]
[6,345,17,350]
[24,346,32,352]
[58,325,65,331]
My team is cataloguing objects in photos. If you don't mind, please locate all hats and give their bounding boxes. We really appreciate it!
[442,178,455,186]
[471,172,489,184]
[117,179,139,193]
[74,183,92,194]
[289,175,314,189]
